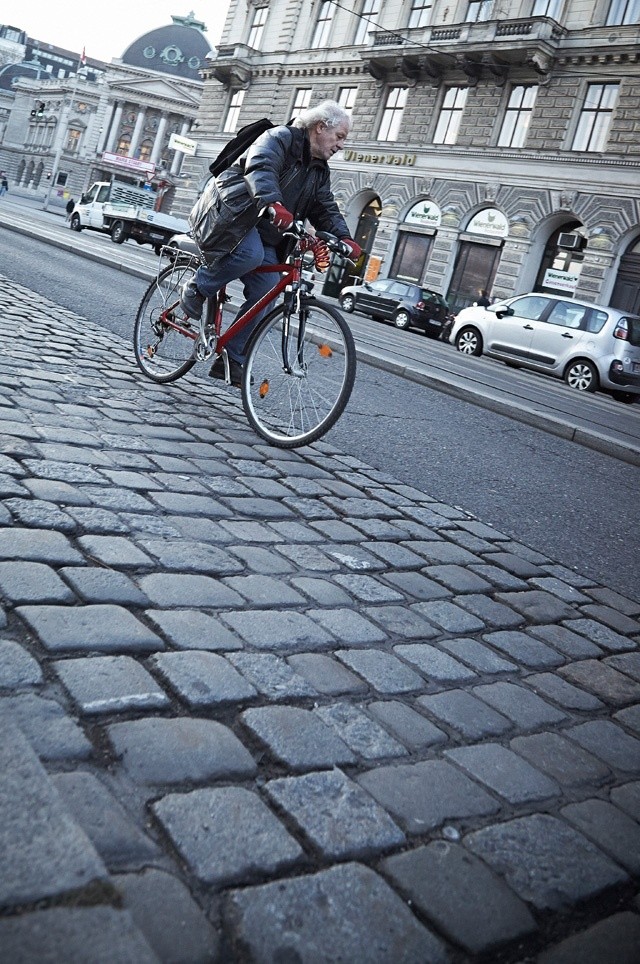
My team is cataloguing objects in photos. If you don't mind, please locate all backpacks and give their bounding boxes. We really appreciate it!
[211,119,302,179]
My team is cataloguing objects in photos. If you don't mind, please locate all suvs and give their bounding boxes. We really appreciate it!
[448,292,640,405]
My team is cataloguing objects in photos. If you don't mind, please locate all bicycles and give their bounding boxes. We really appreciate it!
[133,212,369,448]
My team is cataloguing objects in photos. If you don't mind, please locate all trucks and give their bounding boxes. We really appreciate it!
[68,174,192,256]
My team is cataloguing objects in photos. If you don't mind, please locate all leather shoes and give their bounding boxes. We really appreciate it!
[208,356,255,385]
[178,273,204,322]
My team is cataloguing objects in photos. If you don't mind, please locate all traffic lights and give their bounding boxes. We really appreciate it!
[47,172,52,180]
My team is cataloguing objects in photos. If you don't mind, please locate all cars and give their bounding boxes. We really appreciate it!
[339,279,449,340]
[168,231,202,264]
[283,256,315,294]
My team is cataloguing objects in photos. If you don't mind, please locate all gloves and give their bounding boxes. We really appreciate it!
[267,202,295,234]
[339,237,361,262]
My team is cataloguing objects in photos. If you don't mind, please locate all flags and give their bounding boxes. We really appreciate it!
[80,46,87,65]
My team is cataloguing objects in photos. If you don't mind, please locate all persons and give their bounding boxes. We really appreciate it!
[64,198,75,223]
[473,288,491,306]
[180,100,362,385]
[0,177,8,197]
